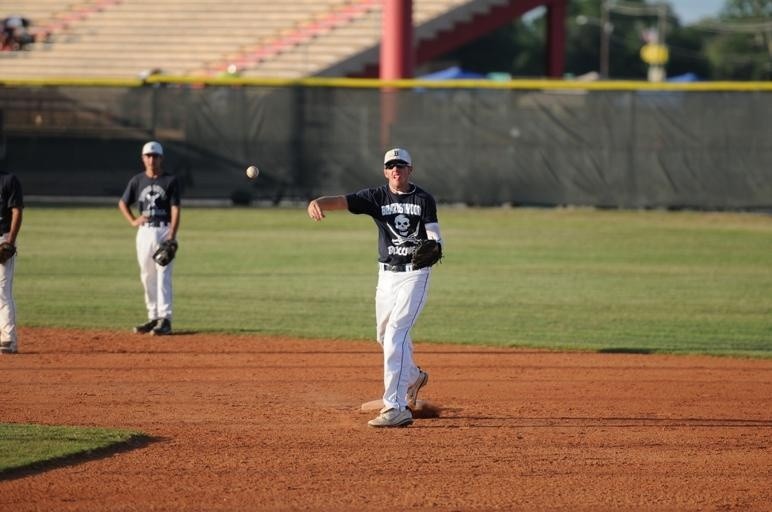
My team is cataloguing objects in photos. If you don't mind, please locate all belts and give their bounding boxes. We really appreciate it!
[384,265,420,272]
[142,221,168,227]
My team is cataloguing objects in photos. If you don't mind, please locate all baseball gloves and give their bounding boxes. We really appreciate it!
[412,239,442,270]
[0,242,16,264]
[152,240,178,266]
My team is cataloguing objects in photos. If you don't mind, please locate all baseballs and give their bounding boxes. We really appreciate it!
[246,166,259,179]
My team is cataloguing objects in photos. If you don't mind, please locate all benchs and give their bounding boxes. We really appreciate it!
[0,2,513,79]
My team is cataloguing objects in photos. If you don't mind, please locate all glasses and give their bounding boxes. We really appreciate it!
[387,161,406,169]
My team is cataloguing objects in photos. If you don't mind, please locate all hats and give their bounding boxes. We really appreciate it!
[141,141,164,156]
[384,148,412,166]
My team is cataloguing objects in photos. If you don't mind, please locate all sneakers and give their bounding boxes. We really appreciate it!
[367,366,428,427]
[1,341,16,353]
[133,319,171,335]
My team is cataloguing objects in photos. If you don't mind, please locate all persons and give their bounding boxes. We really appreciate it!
[306,147,441,427]
[1,156,26,354]
[1,15,36,52]
[117,139,181,336]
